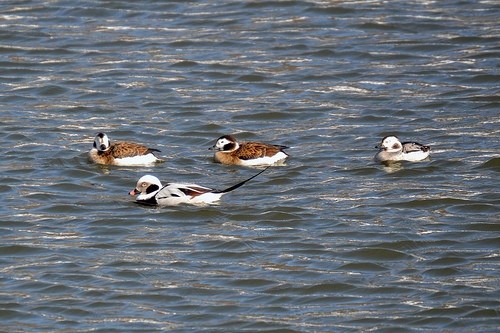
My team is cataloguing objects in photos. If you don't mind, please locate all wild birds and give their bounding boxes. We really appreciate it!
[129,163,273,207]
[249,134,251,135]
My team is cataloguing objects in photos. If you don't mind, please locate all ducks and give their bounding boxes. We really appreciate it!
[374,135,431,163]
[207,135,291,168]
[89,133,162,168]
